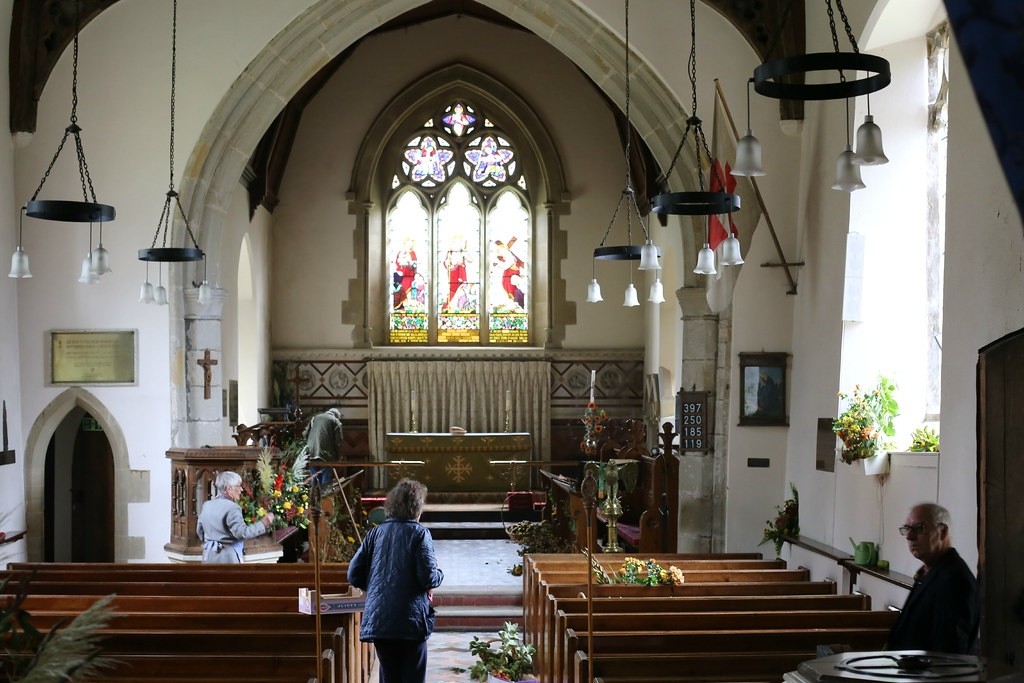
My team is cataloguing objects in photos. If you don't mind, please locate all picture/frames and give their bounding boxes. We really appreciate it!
[737,350,793,428]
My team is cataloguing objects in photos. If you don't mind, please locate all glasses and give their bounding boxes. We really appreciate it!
[898,523,944,536]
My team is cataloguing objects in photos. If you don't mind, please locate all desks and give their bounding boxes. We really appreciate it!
[781,651,1024,683]
[385,431,533,493]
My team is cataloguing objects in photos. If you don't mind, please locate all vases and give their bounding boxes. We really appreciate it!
[837,451,890,477]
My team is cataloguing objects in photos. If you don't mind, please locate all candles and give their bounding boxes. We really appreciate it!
[410,390,416,412]
[590,367,597,403]
[504,390,511,412]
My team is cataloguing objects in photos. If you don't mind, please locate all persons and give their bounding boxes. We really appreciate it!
[196,470,276,564]
[301,408,344,490]
[348,479,443,683]
[883,503,980,652]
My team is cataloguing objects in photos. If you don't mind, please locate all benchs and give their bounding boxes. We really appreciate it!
[520,551,902,683]
[0,561,376,683]
[579,419,679,553]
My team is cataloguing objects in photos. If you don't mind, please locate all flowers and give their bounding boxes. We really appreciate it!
[757,481,799,557]
[329,488,381,563]
[234,442,313,532]
[508,564,523,577]
[579,403,607,460]
[831,377,899,465]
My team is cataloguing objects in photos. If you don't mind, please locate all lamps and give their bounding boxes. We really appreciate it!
[637,0,745,276]
[8,0,116,285]
[729,0,891,193]
[587,0,667,308]
[139,0,212,309]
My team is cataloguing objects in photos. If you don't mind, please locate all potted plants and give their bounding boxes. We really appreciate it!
[467,621,538,683]
